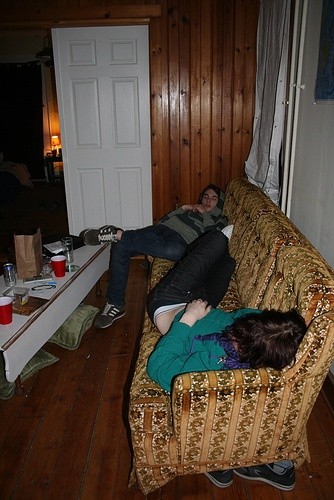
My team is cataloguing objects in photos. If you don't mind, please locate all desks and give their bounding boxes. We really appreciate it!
[46,155,65,187]
[0,241,111,383]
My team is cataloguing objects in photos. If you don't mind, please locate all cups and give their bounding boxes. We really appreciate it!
[51,255,66,277]
[62,237,74,264]
[0,297,13,325]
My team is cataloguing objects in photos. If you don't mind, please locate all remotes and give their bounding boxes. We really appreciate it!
[23,274,53,284]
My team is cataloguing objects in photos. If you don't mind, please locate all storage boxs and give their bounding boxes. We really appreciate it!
[3,286,30,305]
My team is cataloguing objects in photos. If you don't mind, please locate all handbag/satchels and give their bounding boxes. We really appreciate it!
[14,227,43,280]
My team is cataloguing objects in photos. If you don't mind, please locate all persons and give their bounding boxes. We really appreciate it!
[147,228,307,392]
[82,184,229,328]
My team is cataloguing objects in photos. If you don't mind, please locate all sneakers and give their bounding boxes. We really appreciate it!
[79,224,125,246]
[95,302,127,328]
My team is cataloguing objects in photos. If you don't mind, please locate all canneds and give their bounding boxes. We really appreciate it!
[3,263,16,287]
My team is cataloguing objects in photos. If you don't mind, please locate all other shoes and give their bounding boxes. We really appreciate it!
[204,470,234,488]
[233,460,296,491]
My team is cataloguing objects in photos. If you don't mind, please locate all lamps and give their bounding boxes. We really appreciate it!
[50,135,60,157]
[35,34,53,59]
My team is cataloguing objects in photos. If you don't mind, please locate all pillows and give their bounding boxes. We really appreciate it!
[0,303,101,400]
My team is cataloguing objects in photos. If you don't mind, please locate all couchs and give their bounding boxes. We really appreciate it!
[127,176,334,496]
